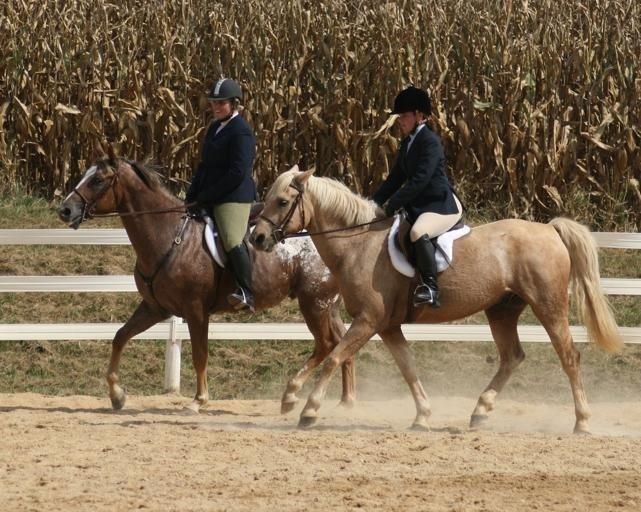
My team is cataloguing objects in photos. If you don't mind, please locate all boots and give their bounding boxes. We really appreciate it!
[411,234,442,309]
[227,245,257,316]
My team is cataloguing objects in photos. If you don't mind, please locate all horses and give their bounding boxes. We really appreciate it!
[57,142,354,415]
[247,164,627,437]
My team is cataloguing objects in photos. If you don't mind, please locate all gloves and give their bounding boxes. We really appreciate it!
[192,192,208,205]
[387,205,396,218]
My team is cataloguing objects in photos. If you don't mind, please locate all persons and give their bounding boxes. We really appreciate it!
[186,76,256,316]
[372,86,463,308]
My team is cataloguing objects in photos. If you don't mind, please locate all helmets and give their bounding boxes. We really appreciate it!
[389,87,431,116]
[208,79,244,102]
